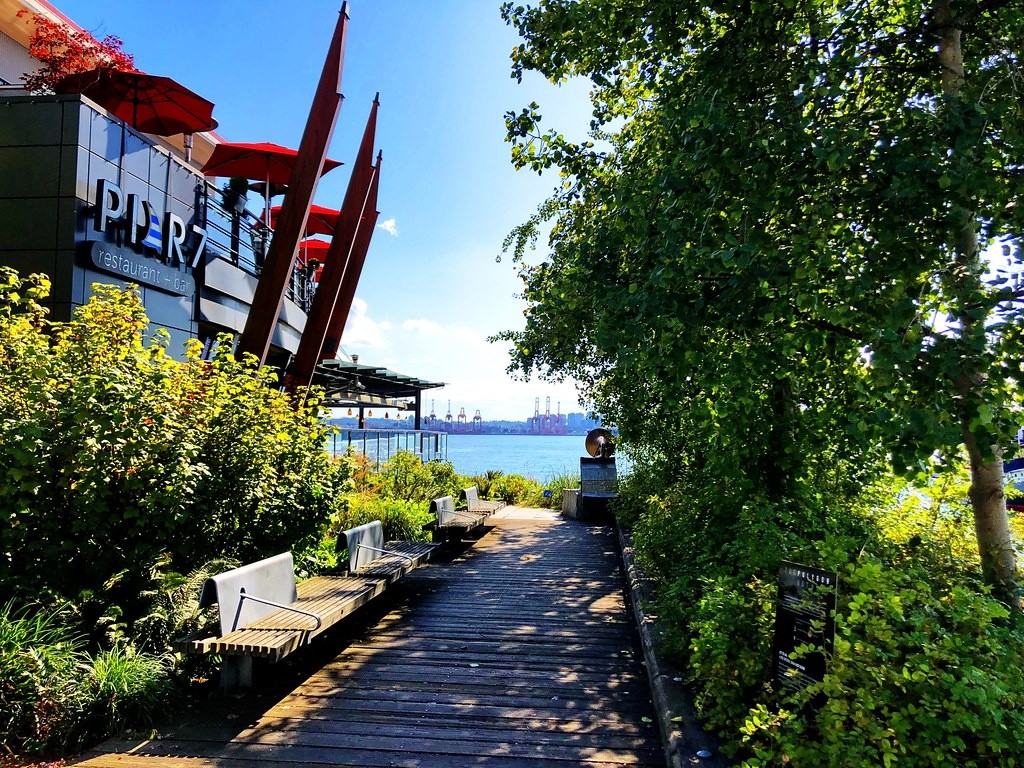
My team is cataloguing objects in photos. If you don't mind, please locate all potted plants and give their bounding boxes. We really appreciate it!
[223,176,249,215]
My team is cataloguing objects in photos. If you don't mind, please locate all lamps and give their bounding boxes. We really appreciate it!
[356,407,359,419]
[405,411,408,420]
[362,417,365,422]
[397,408,400,419]
[347,408,352,416]
[384,408,389,419]
[367,407,372,417]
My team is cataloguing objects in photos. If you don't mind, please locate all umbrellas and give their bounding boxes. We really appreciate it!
[52,66,216,138]
[200,141,345,226]
[254,204,341,289]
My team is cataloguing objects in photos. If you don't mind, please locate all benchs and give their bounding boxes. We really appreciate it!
[172,551,386,698]
[335,521,443,584]
[457,486,507,514]
[421,494,490,533]
